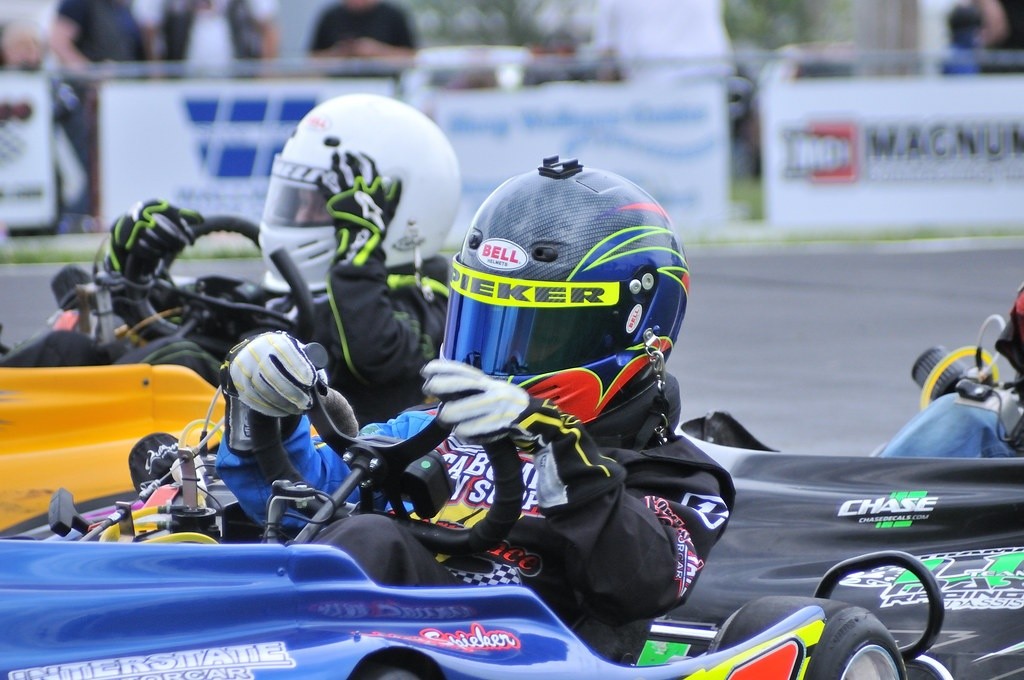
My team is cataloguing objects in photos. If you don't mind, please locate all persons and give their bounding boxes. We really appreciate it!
[0,93,462,389]
[938,0,1008,73]
[0,0,280,118]
[311,0,415,77]
[876,284,1024,457]
[214,155,737,667]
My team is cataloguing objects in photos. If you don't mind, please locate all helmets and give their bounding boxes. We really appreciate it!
[258,93,460,294]
[437,155,693,425]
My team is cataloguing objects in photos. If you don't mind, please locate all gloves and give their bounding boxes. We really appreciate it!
[108,199,205,277]
[314,148,402,263]
[226,328,328,416]
[421,358,625,515]
